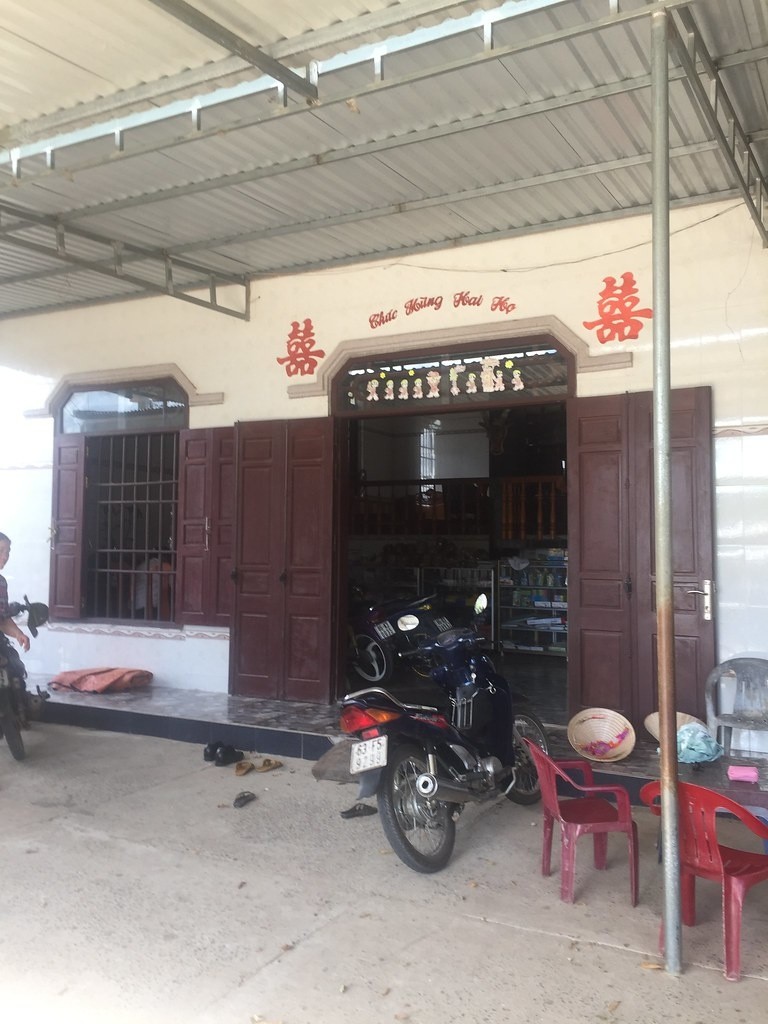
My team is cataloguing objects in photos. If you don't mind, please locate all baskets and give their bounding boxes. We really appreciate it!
[568,708,636,761]
[644,709,707,745]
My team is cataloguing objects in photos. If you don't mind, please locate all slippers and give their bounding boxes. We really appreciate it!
[255,759,283,773]
[234,762,254,776]
[234,791,255,808]
[341,802,377,818]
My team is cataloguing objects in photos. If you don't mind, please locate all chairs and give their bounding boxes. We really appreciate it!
[705,657,768,755]
[521,736,639,908]
[640,779,768,982]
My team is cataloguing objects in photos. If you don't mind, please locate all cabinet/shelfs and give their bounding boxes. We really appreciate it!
[354,559,568,657]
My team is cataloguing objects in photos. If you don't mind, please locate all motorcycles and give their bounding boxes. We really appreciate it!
[348,594,448,689]
[0,595,49,761]
[339,591,552,875]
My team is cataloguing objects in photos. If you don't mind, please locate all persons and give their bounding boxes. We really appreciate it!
[130,545,172,620]
[0,531,31,674]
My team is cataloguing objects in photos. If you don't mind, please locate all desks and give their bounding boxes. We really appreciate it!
[549,729,768,865]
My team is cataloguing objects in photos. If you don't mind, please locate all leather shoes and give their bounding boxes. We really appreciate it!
[203,742,218,762]
[215,744,245,767]
